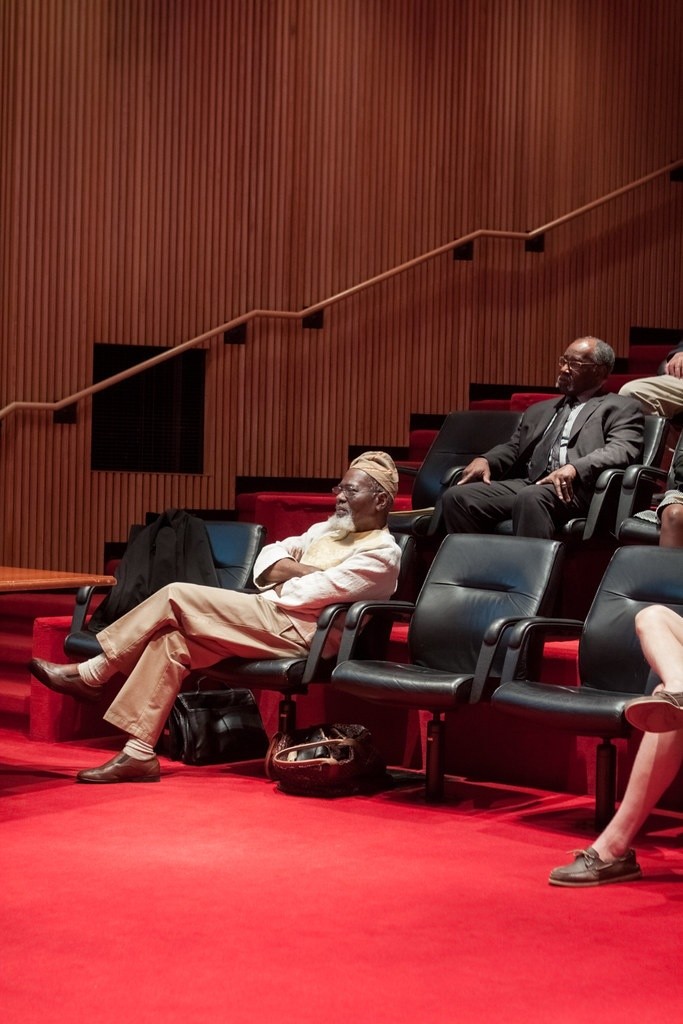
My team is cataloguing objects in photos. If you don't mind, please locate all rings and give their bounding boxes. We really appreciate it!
[561,482,567,488]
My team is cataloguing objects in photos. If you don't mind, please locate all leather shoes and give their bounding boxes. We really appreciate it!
[28,659,100,704]
[78,752,161,784]
[625,690,683,733]
[548,846,639,886]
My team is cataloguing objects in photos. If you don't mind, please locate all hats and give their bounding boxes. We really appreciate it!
[350,450,399,501]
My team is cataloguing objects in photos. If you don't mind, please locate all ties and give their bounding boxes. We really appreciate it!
[530,398,575,482]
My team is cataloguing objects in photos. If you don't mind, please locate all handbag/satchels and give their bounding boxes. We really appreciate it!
[273,726,368,790]
[172,676,267,765]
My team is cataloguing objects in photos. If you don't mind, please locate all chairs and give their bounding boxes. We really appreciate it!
[492,543,682,833]
[615,465,670,540]
[385,410,525,530]
[333,532,564,803]
[64,522,266,656]
[205,532,425,738]
[500,416,671,547]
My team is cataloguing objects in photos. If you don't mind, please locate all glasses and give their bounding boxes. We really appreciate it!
[558,357,599,371]
[332,485,383,496]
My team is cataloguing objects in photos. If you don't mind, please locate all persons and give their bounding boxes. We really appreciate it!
[617,341,683,417]
[548,605,683,885]
[656,439,683,546]
[30,451,402,781]
[442,338,645,539]
